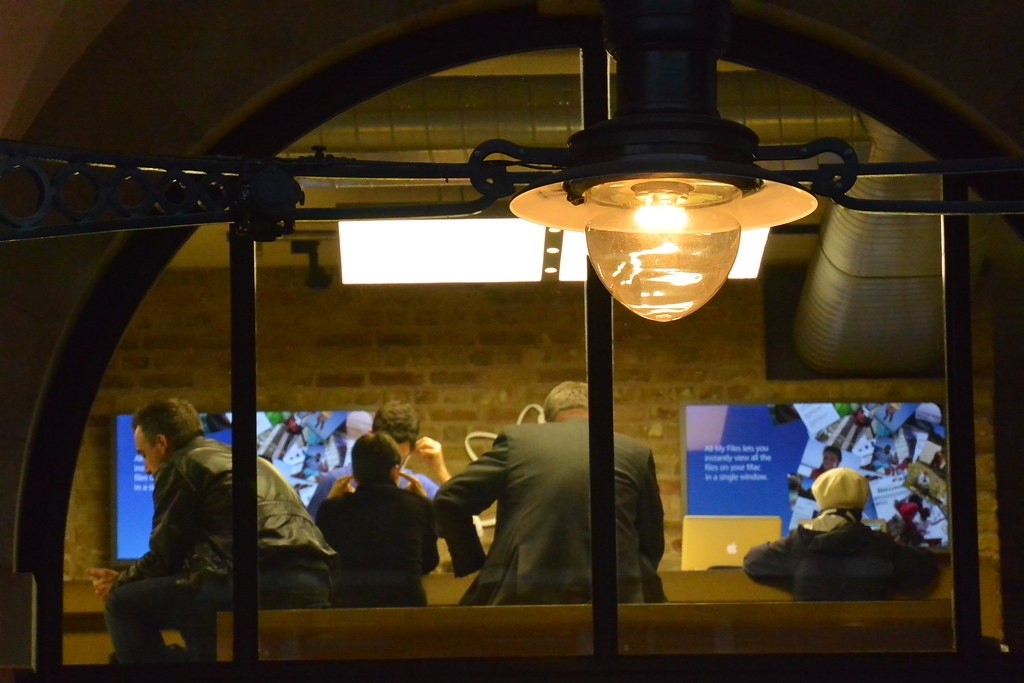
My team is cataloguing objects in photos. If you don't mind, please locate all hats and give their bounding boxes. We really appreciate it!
[811,467,869,512]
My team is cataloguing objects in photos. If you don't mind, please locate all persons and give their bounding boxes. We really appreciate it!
[432,380,667,607]
[307,399,484,543]
[315,430,440,607]
[916,507,932,536]
[293,484,301,497]
[810,445,843,479]
[873,444,892,463]
[85,395,336,662]
[898,494,923,525]
[291,453,328,483]
[742,468,941,601]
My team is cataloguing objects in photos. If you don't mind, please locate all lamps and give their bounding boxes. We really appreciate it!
[508,0,820,326]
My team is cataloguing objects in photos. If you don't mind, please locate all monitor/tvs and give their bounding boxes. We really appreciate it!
[677,398,951,560]
[109,411,375,567]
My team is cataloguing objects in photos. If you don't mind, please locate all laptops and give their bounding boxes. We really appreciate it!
[796,517,889,537]
[680,515,781,570]
